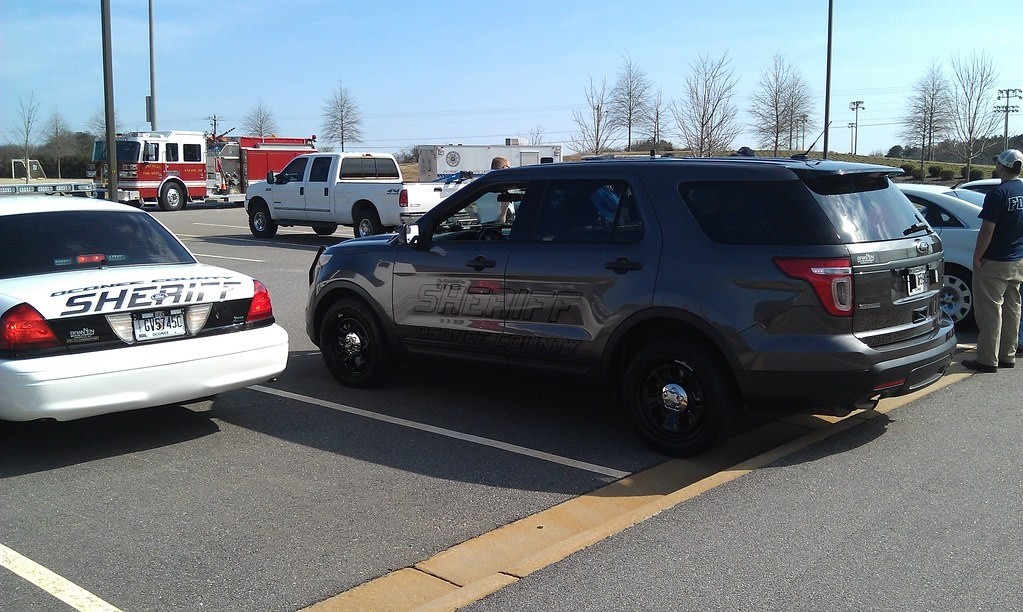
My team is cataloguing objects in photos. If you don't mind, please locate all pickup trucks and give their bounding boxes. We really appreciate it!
[243,151,476,239]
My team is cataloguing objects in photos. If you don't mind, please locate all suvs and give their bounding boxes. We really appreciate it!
[304,151,959,458]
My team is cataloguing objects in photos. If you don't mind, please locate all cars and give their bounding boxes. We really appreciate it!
[0,193,290,423]
[896,177,1022,330]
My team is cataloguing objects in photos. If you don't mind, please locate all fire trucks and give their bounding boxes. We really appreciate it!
[86,114,319,213]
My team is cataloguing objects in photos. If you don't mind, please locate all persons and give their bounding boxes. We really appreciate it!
[463,157,515,228]
[961,148,1023,373]
[990,154,1003,179]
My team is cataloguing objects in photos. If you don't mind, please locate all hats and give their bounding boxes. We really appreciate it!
[993,149,1023,168]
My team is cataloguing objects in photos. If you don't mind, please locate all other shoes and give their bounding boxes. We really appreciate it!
[961,359,997,373]
[998,361,1015,368]
